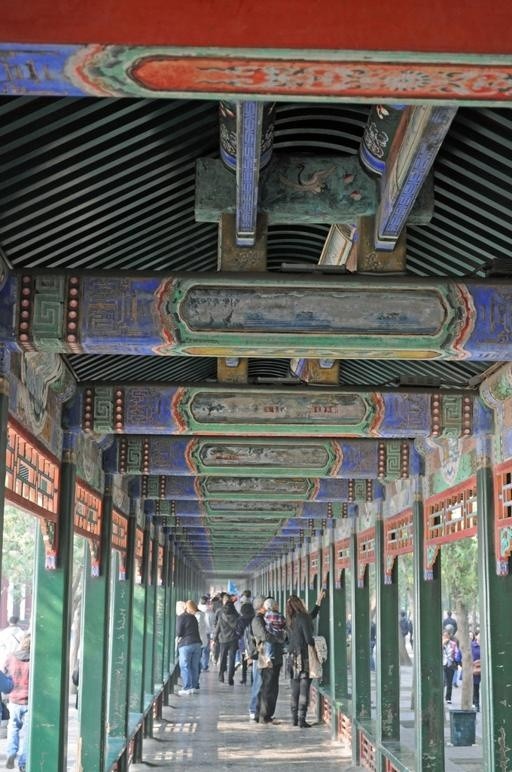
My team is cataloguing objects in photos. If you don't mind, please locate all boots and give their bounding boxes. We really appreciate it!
[240,668,246,686]
[291,704,311,728]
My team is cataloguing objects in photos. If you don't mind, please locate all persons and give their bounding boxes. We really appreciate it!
[345,614,352,672]
[400,608,413,664]
[2,616,32,770]
[442,610,482,712]
[370,615,377,672]
[172,586,326,729]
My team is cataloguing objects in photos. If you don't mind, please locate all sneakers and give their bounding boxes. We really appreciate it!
[177,687,198,695]
[4,754,15,770]
[251,716,281,725]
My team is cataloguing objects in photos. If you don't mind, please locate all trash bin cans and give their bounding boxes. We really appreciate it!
[450,709,476,747]
